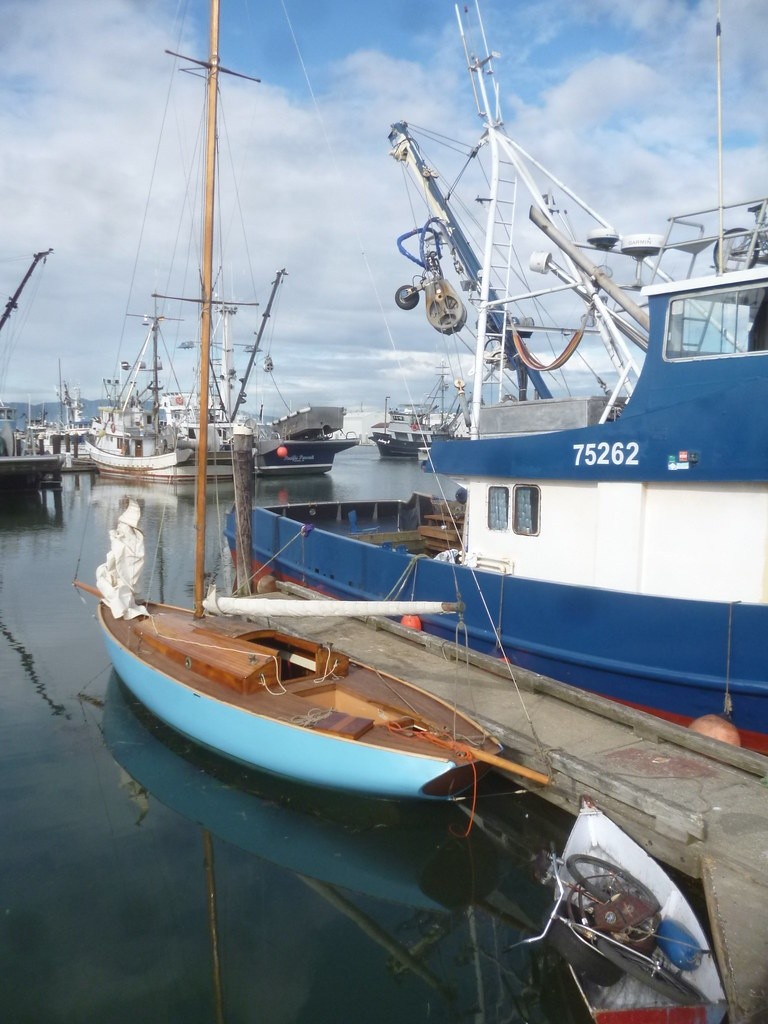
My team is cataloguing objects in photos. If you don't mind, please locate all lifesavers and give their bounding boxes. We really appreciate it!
[111,423,115,433]
[412,424,418,430]
[176,397,183,405]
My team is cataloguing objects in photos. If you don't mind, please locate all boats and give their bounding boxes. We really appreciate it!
[503,792,730,1024]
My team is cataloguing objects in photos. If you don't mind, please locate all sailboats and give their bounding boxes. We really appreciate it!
[0,0,768,750]
[71,0,551,810]
[74,658,498,1024]
